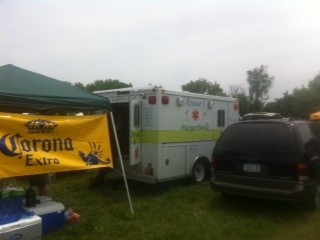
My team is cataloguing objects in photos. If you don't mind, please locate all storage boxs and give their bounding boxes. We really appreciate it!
[0,195,66,240]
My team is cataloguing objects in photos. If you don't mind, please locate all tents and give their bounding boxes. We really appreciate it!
[0,64,134,216]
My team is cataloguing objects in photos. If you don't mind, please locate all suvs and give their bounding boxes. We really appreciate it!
[211,118,320,210]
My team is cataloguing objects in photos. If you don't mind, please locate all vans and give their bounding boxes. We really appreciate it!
[92,87,282,185]
